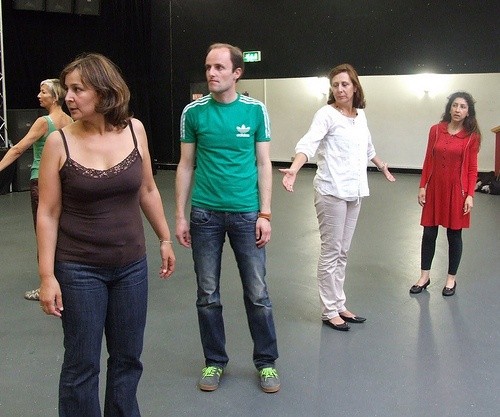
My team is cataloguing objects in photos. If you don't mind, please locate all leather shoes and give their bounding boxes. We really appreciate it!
[339,313,367,325]
[322,317,349,331]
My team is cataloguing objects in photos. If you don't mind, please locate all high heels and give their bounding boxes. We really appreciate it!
[443,280,457,297]
[410,278,431,293]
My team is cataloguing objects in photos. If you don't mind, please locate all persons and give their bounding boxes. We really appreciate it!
[176,42,279,392]
[34,51,177,417]
[280,62,397,330]
[409,92,482,296]
[0,78,74,300]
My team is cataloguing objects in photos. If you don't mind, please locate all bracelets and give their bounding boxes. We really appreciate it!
[257,211,272,221]
[377,162,388,171]
[159,239,173,243]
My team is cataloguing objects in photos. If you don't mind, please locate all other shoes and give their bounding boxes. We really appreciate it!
[198,364,224,392]
[258,364,279,394]
[23,288,42,301]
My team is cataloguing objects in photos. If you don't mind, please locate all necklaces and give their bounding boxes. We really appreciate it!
[334,102,357,124]
